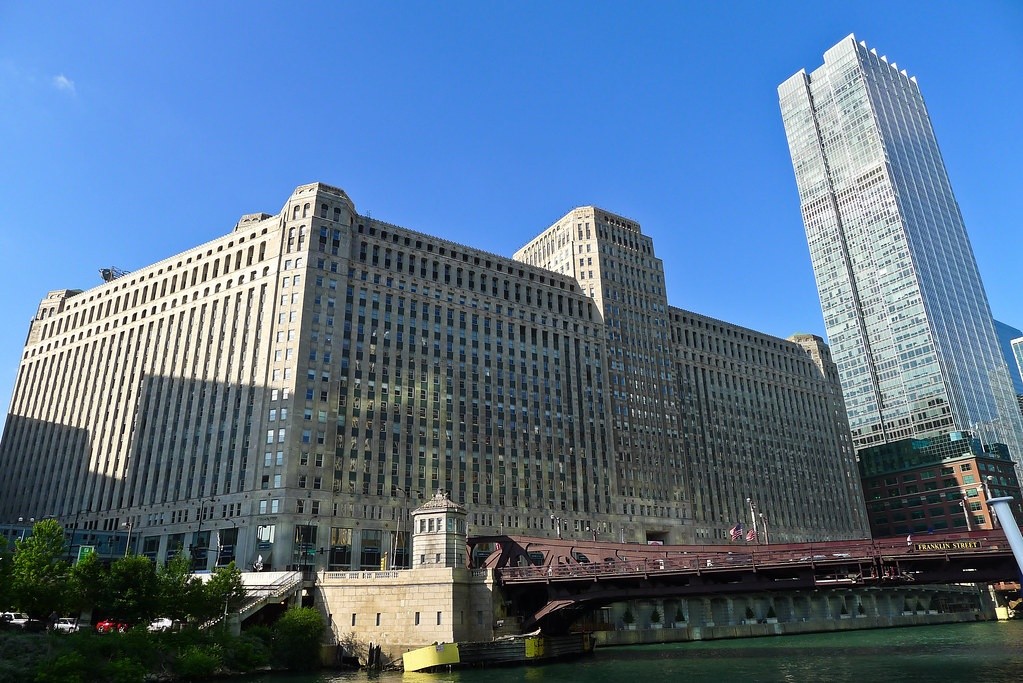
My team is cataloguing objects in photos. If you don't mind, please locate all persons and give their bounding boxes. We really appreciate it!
[907,534,914,553]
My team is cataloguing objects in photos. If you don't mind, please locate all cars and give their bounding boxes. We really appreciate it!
[97,618,128,634]
[0,612,37,628]
[45,617,79,633]
[146,617,178,633]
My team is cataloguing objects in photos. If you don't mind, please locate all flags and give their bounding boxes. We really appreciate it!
[746,528,759,541]
[730,524,743,541]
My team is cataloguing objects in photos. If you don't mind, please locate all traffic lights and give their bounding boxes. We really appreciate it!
[110,539,114,547]
[189,544,193,551]
[320,547,323,555]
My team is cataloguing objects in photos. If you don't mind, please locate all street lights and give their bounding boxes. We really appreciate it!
[977,474,999,529]
[192,499,217,574]
[397,488,421,569]
[959,497,972,531]
[586,527,596,540]
[225,519,236,563]
[941,429,985,452]
[67,508,92,558]
[17,517,35,542]
[760,513,770,544]
[122,522,132,558]
[747,497,760,544]
[551,515,561,539]
[258,526,266,539]
[305,514,318,574]
